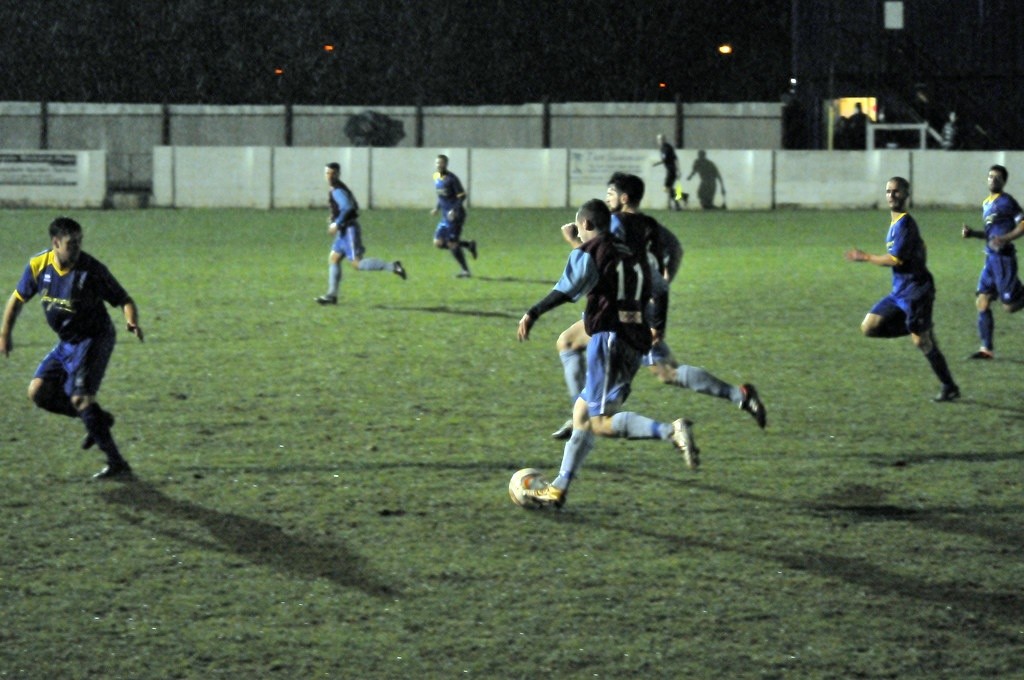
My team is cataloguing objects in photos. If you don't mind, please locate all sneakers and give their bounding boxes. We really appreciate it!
[314,293,337,305]
[468,240,477,260]
[739,383,767,431]
[931,385,961,402]
[670,417,699,470]
[392,260,406,280]
[968,346,993,359]
[455,271,470,277]
[551,419,573,439]
[522,481,566,511]
[81,411,115,450]
[93,461,135,479]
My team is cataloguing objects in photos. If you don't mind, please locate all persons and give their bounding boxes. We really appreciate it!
[962,164,1024,361]
[313,162,408,304]
[517,172,767,509]
[429,154,478,278]
[0,217,145,480]
[844,102,875,149]
[846,176,961,404]
[651,134,682,212]
[0,0,1024,151]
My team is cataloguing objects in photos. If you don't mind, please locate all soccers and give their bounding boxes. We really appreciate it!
[508,467,546,509]
